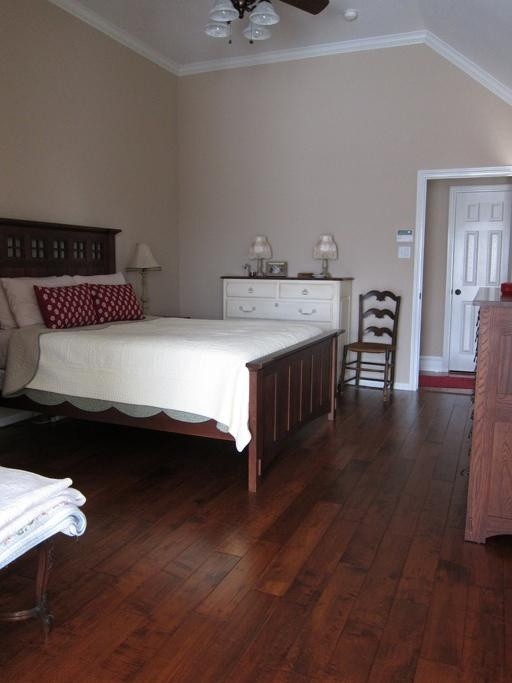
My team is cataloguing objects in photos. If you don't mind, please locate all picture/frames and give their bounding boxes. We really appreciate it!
[264,260,288,276]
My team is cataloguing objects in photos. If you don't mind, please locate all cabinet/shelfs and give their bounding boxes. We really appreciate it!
[461,285,512,546]
[219,274,355,388]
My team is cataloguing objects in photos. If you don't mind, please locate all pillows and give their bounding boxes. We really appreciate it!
[90,282,147,324]
[33,282,103,329]
[0,273,79,328]
[74,270,129,284]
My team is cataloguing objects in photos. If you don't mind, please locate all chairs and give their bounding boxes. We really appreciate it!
[0,536,64,654]
[339,288,402,402]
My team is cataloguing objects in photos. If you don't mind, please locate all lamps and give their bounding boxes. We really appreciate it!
[311,231,339,277]
[201,0,332,46]
[246,233,274,277]
[125,240,163,314]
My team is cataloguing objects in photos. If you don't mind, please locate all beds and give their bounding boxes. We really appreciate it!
[0,214,348,494]
